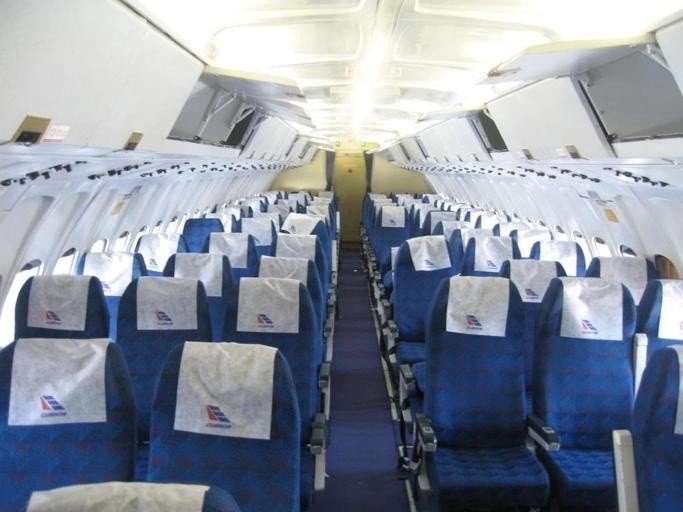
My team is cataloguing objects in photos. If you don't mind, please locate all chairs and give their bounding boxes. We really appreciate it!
[359,186,683,511]
[0,183,337,512]
